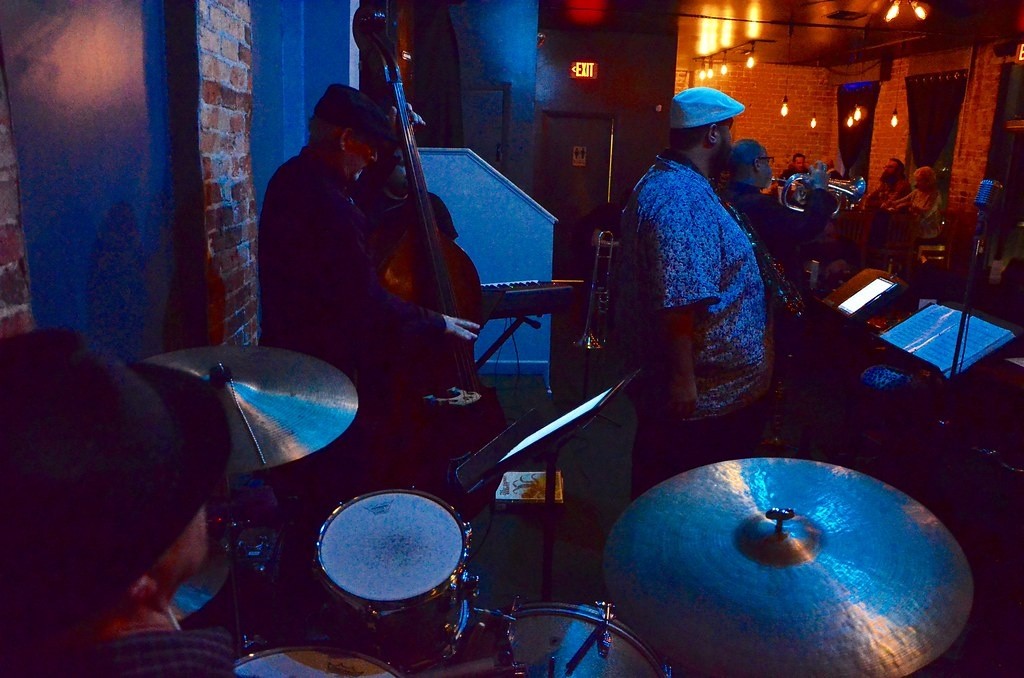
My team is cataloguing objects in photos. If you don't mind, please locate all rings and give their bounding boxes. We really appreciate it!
[823,165,826,171]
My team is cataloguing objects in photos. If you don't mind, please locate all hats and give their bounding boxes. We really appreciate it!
[314,82,397,154]
[0,326,233,657]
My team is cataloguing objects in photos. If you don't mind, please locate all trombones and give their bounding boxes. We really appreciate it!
[573,227,617,419]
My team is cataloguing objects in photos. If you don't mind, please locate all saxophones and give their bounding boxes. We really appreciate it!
[725,202,806,320]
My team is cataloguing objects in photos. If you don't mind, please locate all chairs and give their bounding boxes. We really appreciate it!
[836,210,959,280]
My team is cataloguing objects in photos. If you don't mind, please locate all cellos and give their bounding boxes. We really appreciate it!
[349,0,509,525]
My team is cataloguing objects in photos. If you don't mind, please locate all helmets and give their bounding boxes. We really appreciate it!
[669,87,746,129]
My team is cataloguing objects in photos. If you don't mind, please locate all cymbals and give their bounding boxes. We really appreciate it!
[601,454,976,677]
[154,341,360,478]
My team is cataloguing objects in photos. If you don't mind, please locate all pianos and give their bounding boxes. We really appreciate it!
[475,277,572,374]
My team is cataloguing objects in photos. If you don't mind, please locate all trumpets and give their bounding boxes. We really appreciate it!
[771,172,866,221]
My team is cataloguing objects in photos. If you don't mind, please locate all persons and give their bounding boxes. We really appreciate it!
[777,153,812,187]
[719,138,839,425]
[612,86,772,506]
[255,82,482,645]
[853,157,948,276]
[0,325,267,678]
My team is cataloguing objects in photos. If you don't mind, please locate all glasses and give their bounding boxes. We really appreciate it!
[882,165,895,170]
[752,157,775,167]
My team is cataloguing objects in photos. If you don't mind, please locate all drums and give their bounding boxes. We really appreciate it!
[438,600,668,677]
[310,486,473,672]
[231,644,400,678]
